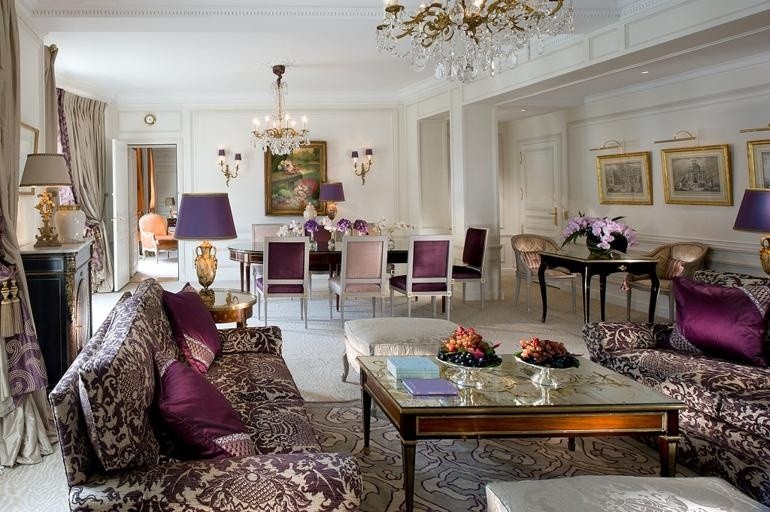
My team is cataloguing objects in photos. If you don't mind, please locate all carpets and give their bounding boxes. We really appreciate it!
[305,399,701,512]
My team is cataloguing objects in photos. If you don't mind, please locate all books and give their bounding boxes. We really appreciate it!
[385,356,459,398]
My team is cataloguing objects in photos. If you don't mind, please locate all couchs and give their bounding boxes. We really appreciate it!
[582,270,770,508]
[48,279,364,512]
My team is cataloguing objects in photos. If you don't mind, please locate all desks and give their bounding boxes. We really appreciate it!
[538,251,659,323]
[21,239,95,391]
[229,248,445,313]
[208,289,257,328]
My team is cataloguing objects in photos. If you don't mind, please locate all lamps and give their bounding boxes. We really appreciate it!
[352,149,372,185]
[21,154,72,247]
[165,198,174,214]
[320,182,346,221]
[733,188,770,275]
[174,193,237,292]
[250,64,310,156]
[376,0,575,83]
[219,150,241,187]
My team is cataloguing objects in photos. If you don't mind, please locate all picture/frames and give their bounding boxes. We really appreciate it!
[264,140,327,216]
[661,144,733,206]
[21,123,39,195]
[747,140,770,189]
[596,151,653,205]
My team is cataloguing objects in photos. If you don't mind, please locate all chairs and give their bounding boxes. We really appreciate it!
[511,234,576,313]
[139,213,177,264]
[624,242,708,323]
[253,224,489,330]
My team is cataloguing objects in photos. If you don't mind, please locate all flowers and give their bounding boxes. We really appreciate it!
[561,210,640,250]
[338,218,351,232]
[305,220,318,232]
[353,220,368,235]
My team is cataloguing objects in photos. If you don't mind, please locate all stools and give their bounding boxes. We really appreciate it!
[341,317,458,382]
[485,477,770,512]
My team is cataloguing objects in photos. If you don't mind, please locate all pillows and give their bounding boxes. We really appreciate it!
[153,351,255,461]
[162,283,222,376]
[664,276,770,368]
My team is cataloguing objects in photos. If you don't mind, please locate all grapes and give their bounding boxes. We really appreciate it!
[437,325,502,369]
[518,335,576,370]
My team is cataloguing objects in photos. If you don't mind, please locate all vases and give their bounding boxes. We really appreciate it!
[586,238,611,256]
